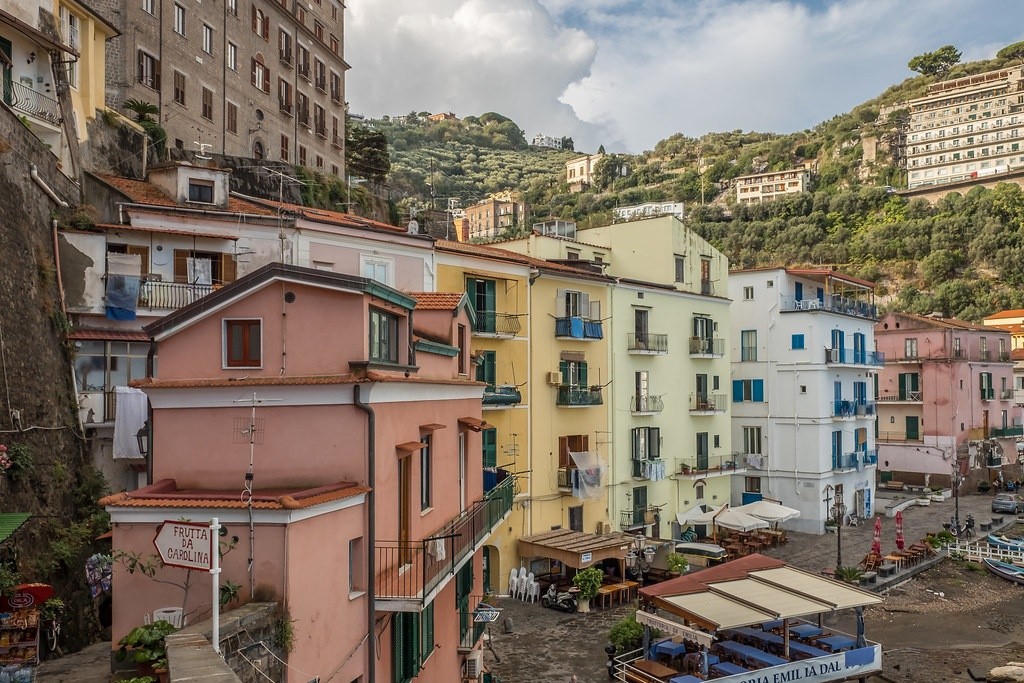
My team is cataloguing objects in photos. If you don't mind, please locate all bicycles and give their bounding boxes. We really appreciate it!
[46,610,62,653]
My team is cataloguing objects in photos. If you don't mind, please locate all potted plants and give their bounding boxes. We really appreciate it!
[921,530,955,553]
[114,620,180,683]
[572,568,603,613]
[824,518,837,533]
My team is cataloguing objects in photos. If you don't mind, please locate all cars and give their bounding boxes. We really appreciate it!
[991,494,1024,515]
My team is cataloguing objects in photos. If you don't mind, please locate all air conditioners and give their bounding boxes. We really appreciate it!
[546,372,563,384]
[825,349,839,364]
[466,650,484,679]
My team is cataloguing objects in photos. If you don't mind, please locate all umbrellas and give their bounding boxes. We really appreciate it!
[678,500,800,534]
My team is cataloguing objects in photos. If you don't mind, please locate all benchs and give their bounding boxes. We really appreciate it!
[1011,571,1023,576]
[906,485,926,492]
[879,564,896,577]
[847,513,864,527]
[859,572,877,586]
[980,515,1004,531]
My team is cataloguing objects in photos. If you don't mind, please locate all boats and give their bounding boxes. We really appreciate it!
[987,533,1024,552]
[982,557,1024,584]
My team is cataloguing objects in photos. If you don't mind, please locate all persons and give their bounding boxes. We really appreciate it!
[993,476,1022,496]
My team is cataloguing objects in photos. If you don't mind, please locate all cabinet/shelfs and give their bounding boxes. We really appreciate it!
[0,616,40,666]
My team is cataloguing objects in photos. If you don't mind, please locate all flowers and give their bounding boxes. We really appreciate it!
[0,444,13,474]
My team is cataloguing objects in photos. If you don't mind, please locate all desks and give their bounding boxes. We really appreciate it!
[883,544,927,574]
[800,299,812,310]
[820,568,835,579]
[535,573,639,612]
[626,618,858,683]
[706,529,782,564]
[909,390,921,401]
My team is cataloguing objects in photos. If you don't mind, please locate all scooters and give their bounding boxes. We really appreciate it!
[541,583,581,613]
[943,513,977,540]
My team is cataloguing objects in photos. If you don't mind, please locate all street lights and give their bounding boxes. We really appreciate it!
[949,462,964,539]
[830,490,849,582]
[624,530,655,601]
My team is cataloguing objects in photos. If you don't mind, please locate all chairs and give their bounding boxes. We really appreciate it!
[507,567,540,604]
[152,607,188,630]
[856,552,884,573]
[794,298,823,310]
